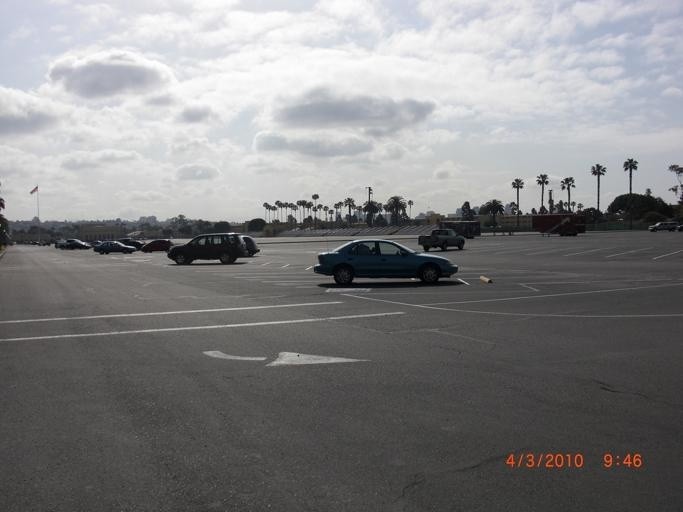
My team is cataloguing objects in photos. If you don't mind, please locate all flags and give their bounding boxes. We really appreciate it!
[29,186,37,194]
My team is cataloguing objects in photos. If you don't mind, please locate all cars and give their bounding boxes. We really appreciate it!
[313,238,459,286]
[167,233,261,265]
[54,237,174,255]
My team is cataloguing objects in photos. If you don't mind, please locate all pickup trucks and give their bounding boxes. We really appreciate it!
[418,228,465,252]
